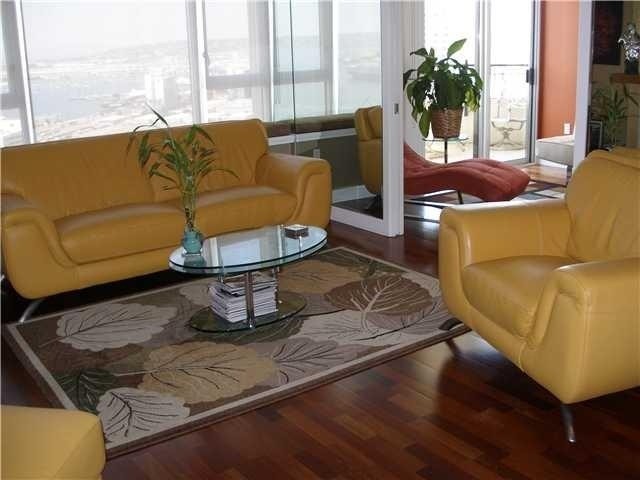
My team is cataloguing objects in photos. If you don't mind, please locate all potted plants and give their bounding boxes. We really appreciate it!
[402,38,484,138]
[126,105,239,253]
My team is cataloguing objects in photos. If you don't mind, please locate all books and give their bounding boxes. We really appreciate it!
[208,271,279,324]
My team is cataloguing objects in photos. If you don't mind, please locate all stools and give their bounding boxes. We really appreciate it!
[1,405,108,480]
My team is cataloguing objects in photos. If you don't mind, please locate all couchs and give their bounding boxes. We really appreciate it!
[436,145,640,444]
[1,117,332,299]
[355,104,531,202]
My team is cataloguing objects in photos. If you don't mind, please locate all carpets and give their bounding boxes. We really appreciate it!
[2,244,471,461]
